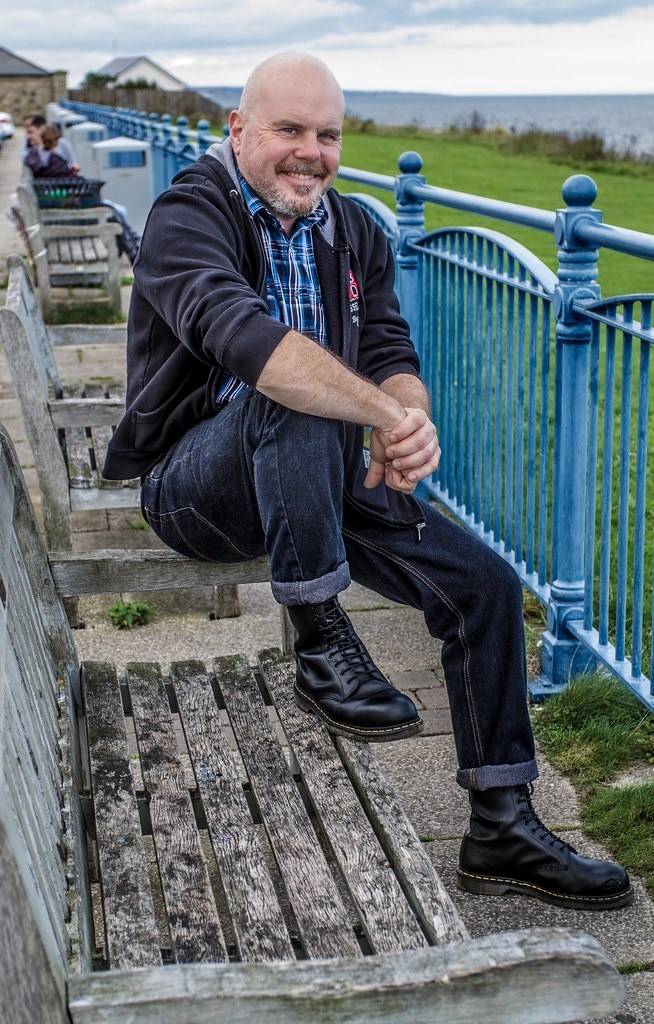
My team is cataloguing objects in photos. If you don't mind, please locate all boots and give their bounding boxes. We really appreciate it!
[289,595,423,739]
[457,783,637,911]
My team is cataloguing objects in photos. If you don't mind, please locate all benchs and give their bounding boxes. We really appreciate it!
[1,161,626,1024]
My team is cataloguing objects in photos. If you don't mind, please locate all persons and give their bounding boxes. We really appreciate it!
[102,49,636,910]
[21,111,142,267]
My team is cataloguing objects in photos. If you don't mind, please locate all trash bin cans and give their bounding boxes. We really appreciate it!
[92,135,155,235]
[44,102,108,180]
